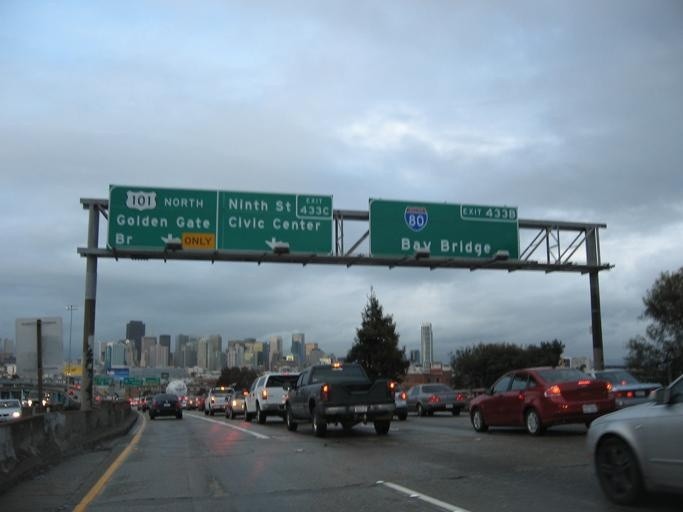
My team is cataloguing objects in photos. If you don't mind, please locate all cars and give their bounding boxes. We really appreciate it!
[467,364,617,437]
[581,372,683,508]
[404,383,467,417]
[148,394,183,419]
[391,381,408,421]
[129,387,208,413]
[589,366,662,406]
[225,391,245,420]
[0,398,23,422]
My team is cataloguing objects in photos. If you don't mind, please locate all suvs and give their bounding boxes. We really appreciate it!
[242,372,300,424]
[27,389,50,407]
[204,386,235,416]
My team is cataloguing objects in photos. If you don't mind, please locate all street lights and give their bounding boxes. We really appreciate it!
[64,304,81,377]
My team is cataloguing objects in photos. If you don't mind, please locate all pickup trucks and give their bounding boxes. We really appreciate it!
[283,364,397,437]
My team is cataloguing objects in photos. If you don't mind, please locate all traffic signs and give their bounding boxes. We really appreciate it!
[366,196,522,262]
[104,183,219,253]
[216,189,336,256]
